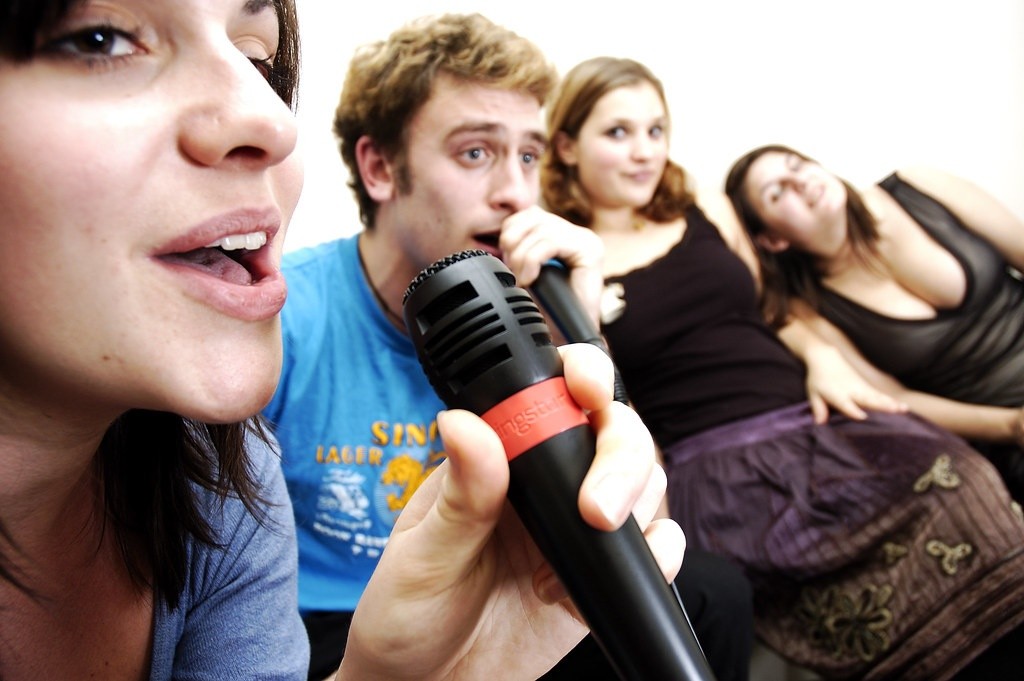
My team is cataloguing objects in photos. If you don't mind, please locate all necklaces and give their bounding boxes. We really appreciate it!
[359,251,405,328]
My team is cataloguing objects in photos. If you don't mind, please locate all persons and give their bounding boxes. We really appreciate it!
[725,146,1024,507]
[545,56,1024,681]
[0,1,686,680]
[260,14,606,681]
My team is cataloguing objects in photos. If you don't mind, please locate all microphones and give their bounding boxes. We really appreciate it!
[528,256,630,405]
[404,250,715,681]
